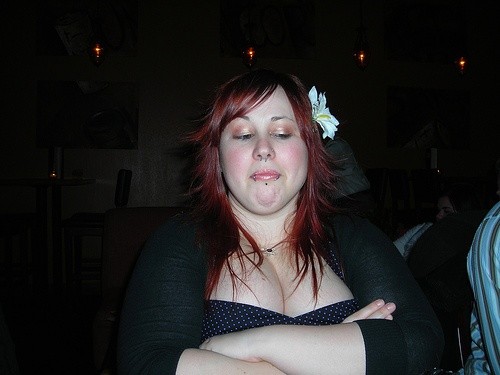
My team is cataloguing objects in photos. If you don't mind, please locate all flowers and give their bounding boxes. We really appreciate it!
[308,85,339,141]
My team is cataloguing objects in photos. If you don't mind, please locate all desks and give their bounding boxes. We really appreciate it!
[0,174,95,298]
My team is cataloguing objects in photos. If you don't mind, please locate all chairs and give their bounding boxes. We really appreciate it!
[0,213,42,298]
[60,169,132,298]
[365,167,433,223]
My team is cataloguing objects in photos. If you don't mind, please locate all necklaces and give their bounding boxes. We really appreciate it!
[244,237,287,255]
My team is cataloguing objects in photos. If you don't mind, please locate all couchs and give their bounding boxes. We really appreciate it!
[93,206,163,375]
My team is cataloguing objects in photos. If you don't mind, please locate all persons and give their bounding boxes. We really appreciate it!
[119,66,445,375]
[321,128,500,375]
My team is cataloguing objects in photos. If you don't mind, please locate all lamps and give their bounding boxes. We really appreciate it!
[242,0,258,69]
[86,0,110,68]
[352,0,371,68]
